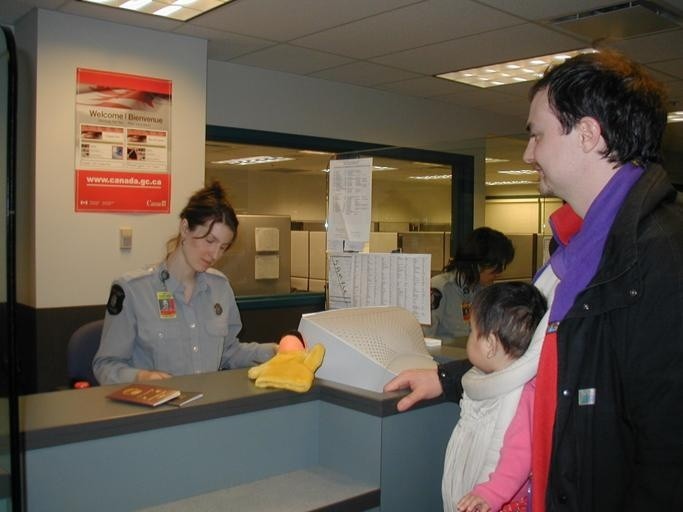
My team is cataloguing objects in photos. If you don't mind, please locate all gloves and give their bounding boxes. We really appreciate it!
[248,344,324,392]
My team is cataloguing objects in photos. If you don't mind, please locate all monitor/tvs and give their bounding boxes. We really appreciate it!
[298,305,439,393]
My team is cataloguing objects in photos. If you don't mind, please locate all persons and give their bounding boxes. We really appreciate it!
[441,281,548,511]
[421,226,515,338]
[385,52,682,510]
[91,179,277,385]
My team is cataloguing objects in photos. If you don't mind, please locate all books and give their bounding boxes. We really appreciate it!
[105,383,181,406]
[166,391,204,406]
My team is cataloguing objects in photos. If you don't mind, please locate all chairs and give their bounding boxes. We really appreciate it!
[68,315,107,390]
